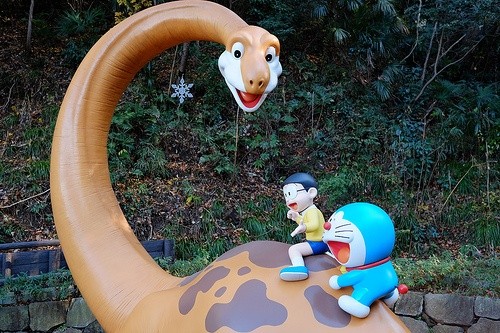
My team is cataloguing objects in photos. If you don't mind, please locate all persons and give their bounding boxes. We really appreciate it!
[278,171,330,283]
